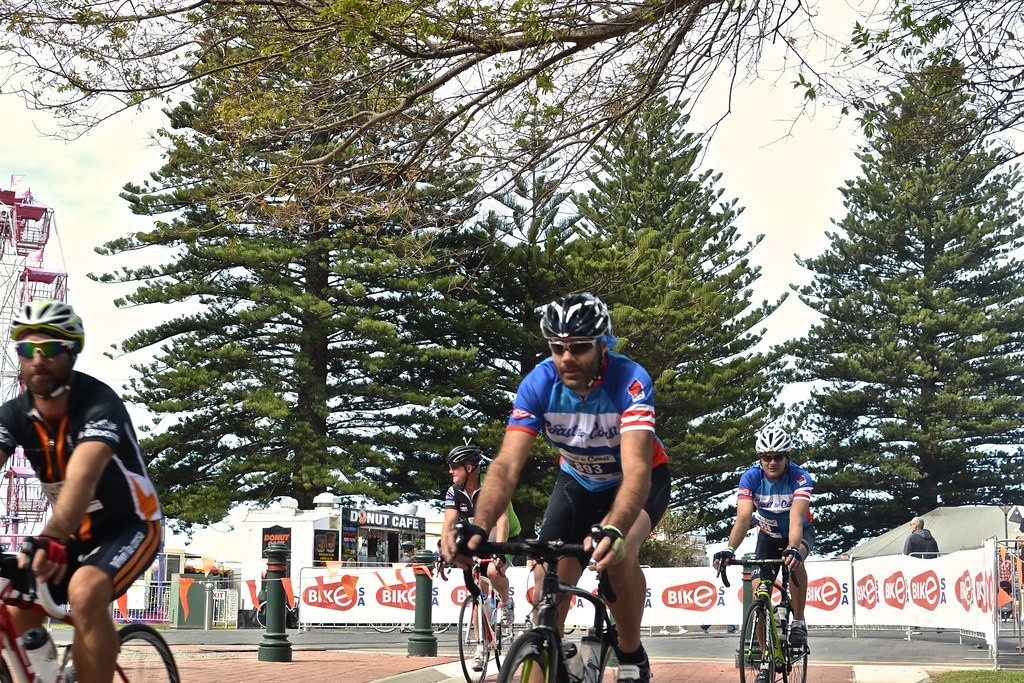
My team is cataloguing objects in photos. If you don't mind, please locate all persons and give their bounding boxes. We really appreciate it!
[1,299,163,683]
[904,517,946,634]
[400,541,416,564]
[439,440,523,671]
[996,553,1013,623]
[712,428,816,683]
[433,539,442,556]
[442,290,672,683]
[1018,534,1024,582]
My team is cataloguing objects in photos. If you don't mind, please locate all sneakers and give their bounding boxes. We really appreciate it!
[659,628,670,635]
[679,629,688,635]
[500,598,514,629]
[472,651,489,670]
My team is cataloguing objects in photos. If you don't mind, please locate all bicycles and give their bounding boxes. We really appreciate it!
[256,596,300,629]
[560,625,578,635]
[441,511,658,683]
[0,531,184,683]
[715,545,812,683]
[370,623,452,634]
[432,551,515,683]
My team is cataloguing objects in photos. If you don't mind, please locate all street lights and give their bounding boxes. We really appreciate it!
[999,502,1013,557]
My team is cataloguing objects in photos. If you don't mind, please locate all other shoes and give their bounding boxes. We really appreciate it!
[789,627,808,644]
[912,630,922,635]
[755,668,770,683]
[617,652,651,683]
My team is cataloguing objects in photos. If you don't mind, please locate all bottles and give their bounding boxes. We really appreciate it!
[773,605,783,636]
[23,625,61,683]
[561,639,585,683]
[775,602,787,640]
[482,594,497,627]
[579,627,602,683]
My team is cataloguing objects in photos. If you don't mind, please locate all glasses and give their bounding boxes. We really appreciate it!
[547,339,599,354]
[448,463,469,470]
[760,455,786,462]
[15,338,78,359]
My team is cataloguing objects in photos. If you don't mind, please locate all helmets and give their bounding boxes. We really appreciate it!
[755,427,791,452]
[540,292,612,339]
[447,446,482,466]
[8,298,84,351]
